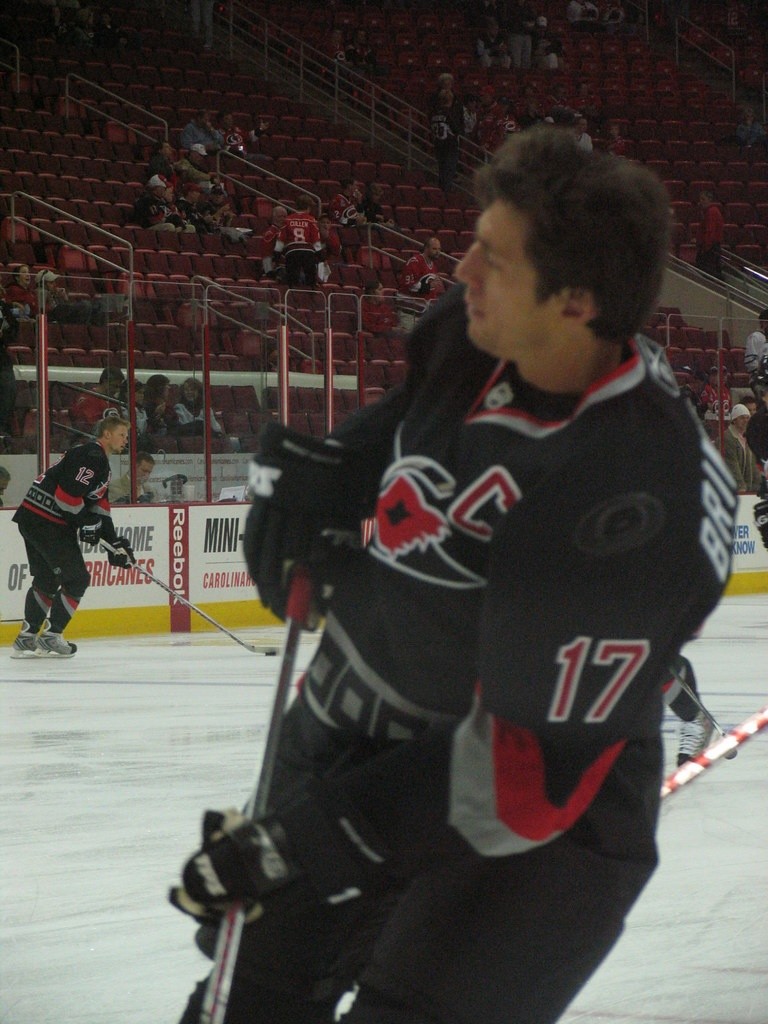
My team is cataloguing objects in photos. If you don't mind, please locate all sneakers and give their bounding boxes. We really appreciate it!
[34,619,77,658]
[676,711,718,769]
[8,619,39,659]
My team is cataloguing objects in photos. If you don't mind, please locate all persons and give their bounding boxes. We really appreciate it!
[169,126,738,1023]
[41,0,131,53]
[736,108,768,151]
[0,296,21,436]
[137,111,271,234]
[70,363,223,453]
[698,366,733,420]
[464,0,620,71]
[0,464,11,508]
[259,177,385,284]
[345,31,377,76]
[11,416,136,656]
[697,190,723,279]
[0,265,108,335]
[658,655,718,766]
[187,0,213,50]
[108,451,158,504]
[423,73,612,191]
[712,309,768,549]
[402,237,447,300]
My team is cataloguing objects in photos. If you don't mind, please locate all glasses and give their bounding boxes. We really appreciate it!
[107,384,121,389]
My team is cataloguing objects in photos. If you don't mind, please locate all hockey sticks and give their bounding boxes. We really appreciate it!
[98,537,280,654]
[199,566,313,1024]
[667,665,738,760]
[660,705,768,801]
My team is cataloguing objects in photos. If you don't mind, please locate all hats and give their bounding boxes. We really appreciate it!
[535,17,548,28]
[191,144,208,156]
[182,182,203,198]
[144,174,167,188]
[120,379,143,394]
[707,361,727,374]
[211,184,224,195]
[34,269,60,282]
[730,403,751,422]
[294,195,317,209]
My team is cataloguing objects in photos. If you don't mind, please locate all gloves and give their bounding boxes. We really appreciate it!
[79,516,102,546]
[747,371,767,397]
[169,781,389,929]
[103,536,135,569]
[241,418,353,631]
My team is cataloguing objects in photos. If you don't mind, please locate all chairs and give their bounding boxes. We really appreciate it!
[0,0,768,459]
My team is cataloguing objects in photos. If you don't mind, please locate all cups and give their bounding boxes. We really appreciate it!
[181,486,195,502]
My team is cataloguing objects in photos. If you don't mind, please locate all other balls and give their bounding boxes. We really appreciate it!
[265,652,276,656]
